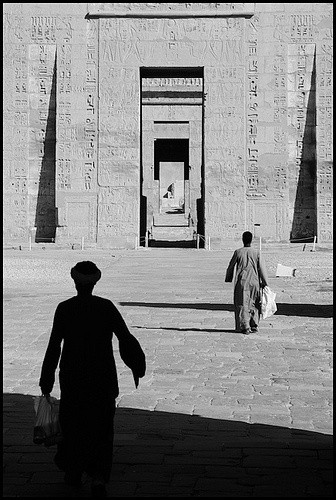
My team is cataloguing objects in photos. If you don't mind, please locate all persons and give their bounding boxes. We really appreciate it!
[38,260,146,490]
[225,230,265,334]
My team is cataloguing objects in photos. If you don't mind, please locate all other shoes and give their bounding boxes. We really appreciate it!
[91,480,114,499]
[242,328,250,335]
[62,467,88,488]
[253,326,258,333]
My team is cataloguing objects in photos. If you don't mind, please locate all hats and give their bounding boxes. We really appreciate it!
[70,260,103,282]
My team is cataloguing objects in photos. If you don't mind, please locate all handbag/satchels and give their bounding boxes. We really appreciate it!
[259,285,278,319]
[31,390,62,446]
